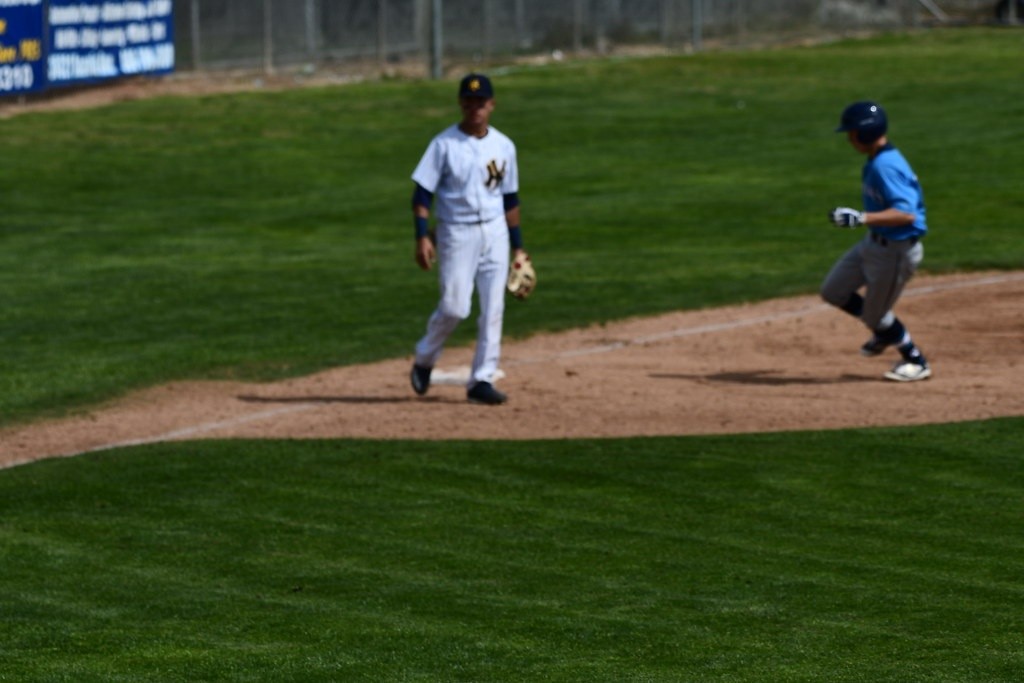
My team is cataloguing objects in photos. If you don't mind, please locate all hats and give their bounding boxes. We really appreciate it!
[459,74,494,99]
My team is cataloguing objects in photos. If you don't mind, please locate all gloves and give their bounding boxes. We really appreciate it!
[830,207,865,228]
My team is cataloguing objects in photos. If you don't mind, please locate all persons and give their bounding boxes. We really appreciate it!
[410,74,537,404]
[820,101,932,382]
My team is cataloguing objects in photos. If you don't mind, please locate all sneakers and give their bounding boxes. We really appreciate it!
[862,335,887,356]
[410,362,434,395]
[885,361,929,381]
[470,381,506,405]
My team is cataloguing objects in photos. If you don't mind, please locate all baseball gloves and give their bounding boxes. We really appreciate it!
[505,248,537,299]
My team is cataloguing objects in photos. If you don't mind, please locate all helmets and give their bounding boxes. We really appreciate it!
[835,102,887,144]
